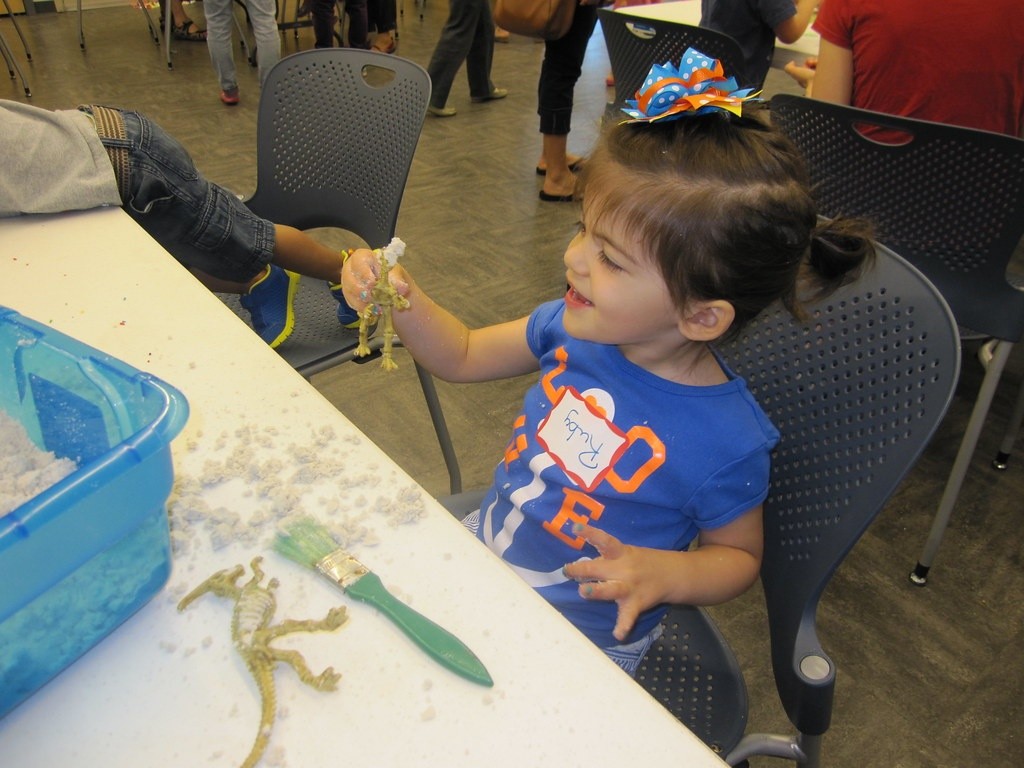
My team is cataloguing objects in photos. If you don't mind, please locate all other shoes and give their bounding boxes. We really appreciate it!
[221,85,240,105]
[426,103,457,116]
[471,88,508,103]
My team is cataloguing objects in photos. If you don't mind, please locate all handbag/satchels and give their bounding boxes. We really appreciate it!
[494,0,575,41]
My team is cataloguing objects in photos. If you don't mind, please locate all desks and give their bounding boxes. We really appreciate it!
[0,204,732,768]
[615,0,821,72]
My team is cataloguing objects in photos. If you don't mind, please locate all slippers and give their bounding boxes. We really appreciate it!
[536,158,586,175]
[539,185,585,202]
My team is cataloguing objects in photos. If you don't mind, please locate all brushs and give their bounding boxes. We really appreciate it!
[270,517,493,687]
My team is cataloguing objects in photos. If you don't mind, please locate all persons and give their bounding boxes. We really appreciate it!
[340,46,876,679]
[0,99,361,347]
[697,0,1024,144]
[159,0,508,116]
[536,0,615,200]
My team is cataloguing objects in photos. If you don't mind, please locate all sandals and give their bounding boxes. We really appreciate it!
[173,19,208,41]
[369,37,396,54]
[160,10,175,32]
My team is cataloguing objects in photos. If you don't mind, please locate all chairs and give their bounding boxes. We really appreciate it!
[212,9,1024,768]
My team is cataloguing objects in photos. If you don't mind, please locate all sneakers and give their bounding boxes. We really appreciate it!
[239,261,301,349]
[327,248,365,327]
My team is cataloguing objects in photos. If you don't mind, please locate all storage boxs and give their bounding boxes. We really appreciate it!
[0,306,190,721]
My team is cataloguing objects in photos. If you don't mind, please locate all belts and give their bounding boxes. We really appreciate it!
[93,105,129,208]
